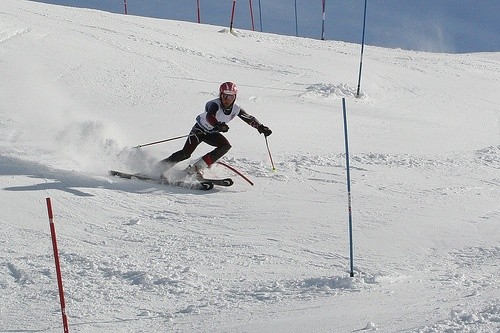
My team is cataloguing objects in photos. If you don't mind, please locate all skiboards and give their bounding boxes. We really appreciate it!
[109,168,234,191]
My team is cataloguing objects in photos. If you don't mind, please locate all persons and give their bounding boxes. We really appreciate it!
[154,82,272,182]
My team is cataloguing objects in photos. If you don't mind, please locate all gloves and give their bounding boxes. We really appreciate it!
[257,123,272,136]
[214,122,229,133]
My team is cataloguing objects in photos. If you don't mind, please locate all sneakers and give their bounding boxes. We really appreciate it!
[189,166,203,181]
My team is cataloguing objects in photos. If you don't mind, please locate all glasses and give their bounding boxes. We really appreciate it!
[220,93,235,100]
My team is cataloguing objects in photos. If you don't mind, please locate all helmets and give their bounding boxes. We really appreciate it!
[219,82,238,95]
